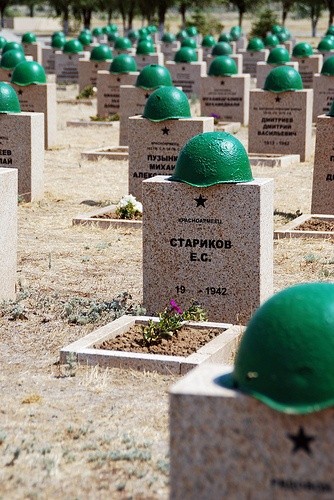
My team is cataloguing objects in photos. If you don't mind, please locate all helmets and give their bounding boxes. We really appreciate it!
[109,54,139,75]
[211,281,334,416]
[264,47,290,65]
[319,54,334,76]
[164,130,255,189]
[262,65,304,93]
[134,64,173,91]
[89,44,115,61]
[161,24,201,64]
[0,36,27,71]
[0,80,22,115]
[245,24,290,52]
[292,40,314,59]
[141,85,192,122]
[207,56,238,78]
[324,95,334,118]
[316,24,334,54]
[50,24,158,55]
[9,60,47,87]
[200,25,241,57]
[21,33,37,43]
